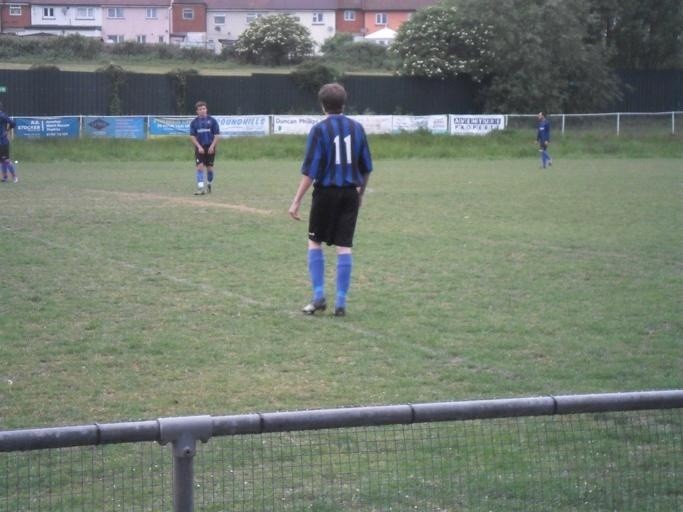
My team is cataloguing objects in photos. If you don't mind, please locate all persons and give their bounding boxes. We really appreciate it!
[0,101,20,183]
[533,111,552,168]
[288,81,373,317]
[188,100,220,195]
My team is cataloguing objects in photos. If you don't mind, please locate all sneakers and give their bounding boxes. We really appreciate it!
[302,298,346,317]
[194,184,213,196]
